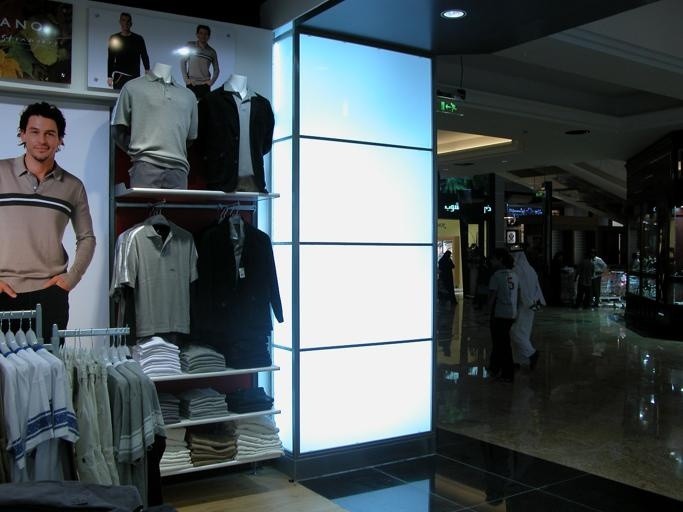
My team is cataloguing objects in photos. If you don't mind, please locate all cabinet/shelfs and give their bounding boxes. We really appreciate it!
[150,364,285,478]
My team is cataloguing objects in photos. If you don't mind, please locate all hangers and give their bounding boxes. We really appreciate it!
[26,310,39,346]
[145,202,169,227]
[217,204,241,225]
[59,327,133,368]
[15,310,28,348]
[0,311,10,355]
[5,311,18,352]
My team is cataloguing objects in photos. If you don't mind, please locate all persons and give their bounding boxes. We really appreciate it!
[574,248,607,309]
[488,244,547,383]
[438,305,456,357]
[106,11,276,192]
[439,251,458,304]
[478,384,544,507]
[0,102,96,348]
